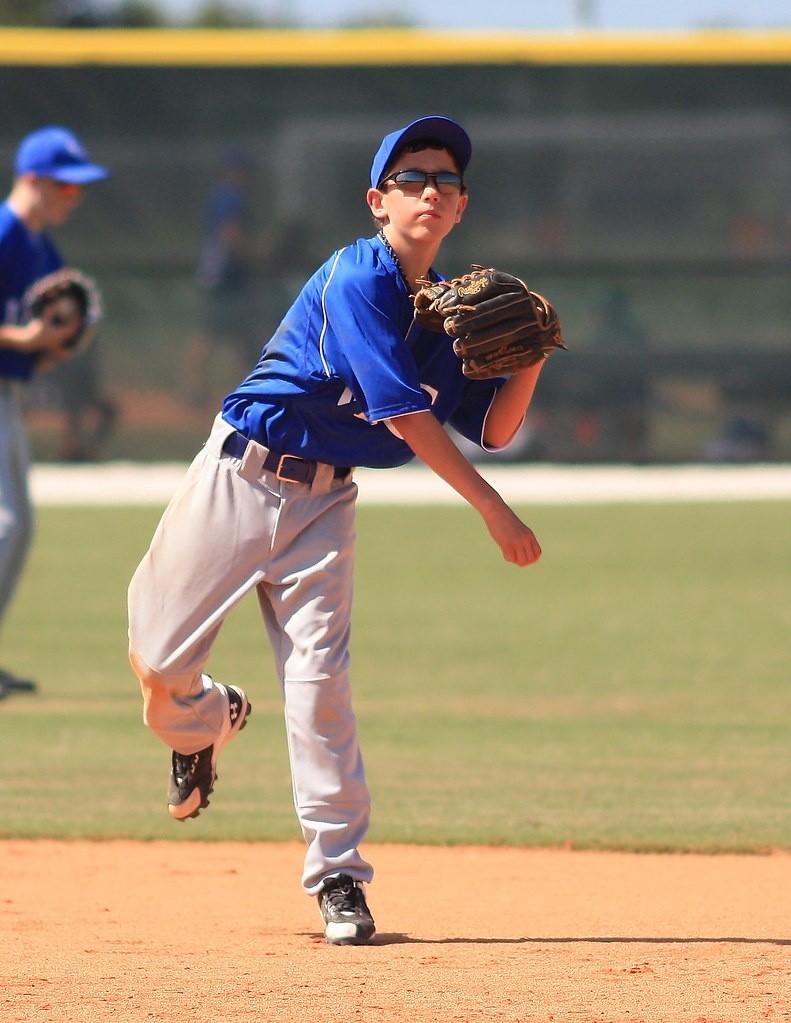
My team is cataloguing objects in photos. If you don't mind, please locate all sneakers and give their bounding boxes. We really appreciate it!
[166,684,252,821]
[320,873,376,944]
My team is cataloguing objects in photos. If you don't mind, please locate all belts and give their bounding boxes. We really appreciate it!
[223,434,349,484]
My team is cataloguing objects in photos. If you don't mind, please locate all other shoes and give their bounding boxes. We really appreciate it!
[0,669,35,700]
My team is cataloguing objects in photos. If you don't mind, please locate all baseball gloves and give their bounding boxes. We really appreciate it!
[406,260,569,384]
[20,263,105,357]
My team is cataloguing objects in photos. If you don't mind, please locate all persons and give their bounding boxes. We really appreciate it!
[126,116,565,947]
[178,146,271,406]
[0,124,111,702]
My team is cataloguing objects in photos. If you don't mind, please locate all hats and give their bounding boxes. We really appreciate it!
[369,116,473,188]
[14,125,110,185]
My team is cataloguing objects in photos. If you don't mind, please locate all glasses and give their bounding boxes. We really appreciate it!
[378,170,468,195]
